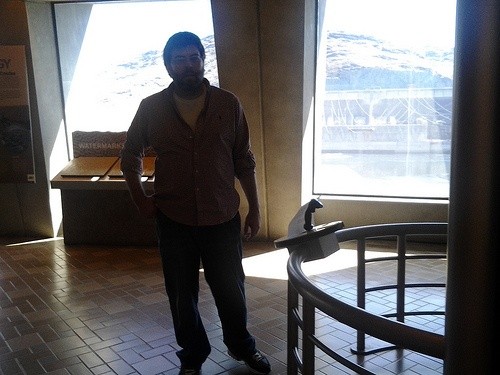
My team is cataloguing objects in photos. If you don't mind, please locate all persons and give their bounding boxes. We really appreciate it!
[120,32,272,375]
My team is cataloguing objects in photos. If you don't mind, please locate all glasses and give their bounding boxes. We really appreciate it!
[170,56,202,64]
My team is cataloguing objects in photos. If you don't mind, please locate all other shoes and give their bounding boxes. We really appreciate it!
[227,347,271,374]
[178,357,206,375]
